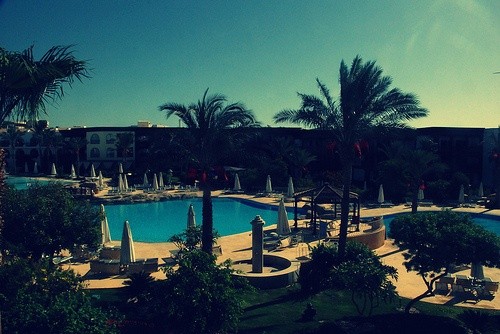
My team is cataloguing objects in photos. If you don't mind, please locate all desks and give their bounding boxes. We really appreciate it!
[169,250,180,258]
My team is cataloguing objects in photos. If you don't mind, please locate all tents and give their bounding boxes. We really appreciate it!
[208,166,247,190]
[292,181,361,237]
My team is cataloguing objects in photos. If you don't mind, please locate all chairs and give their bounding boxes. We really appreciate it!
[435,273,498,304]
[162,258,176,267]
